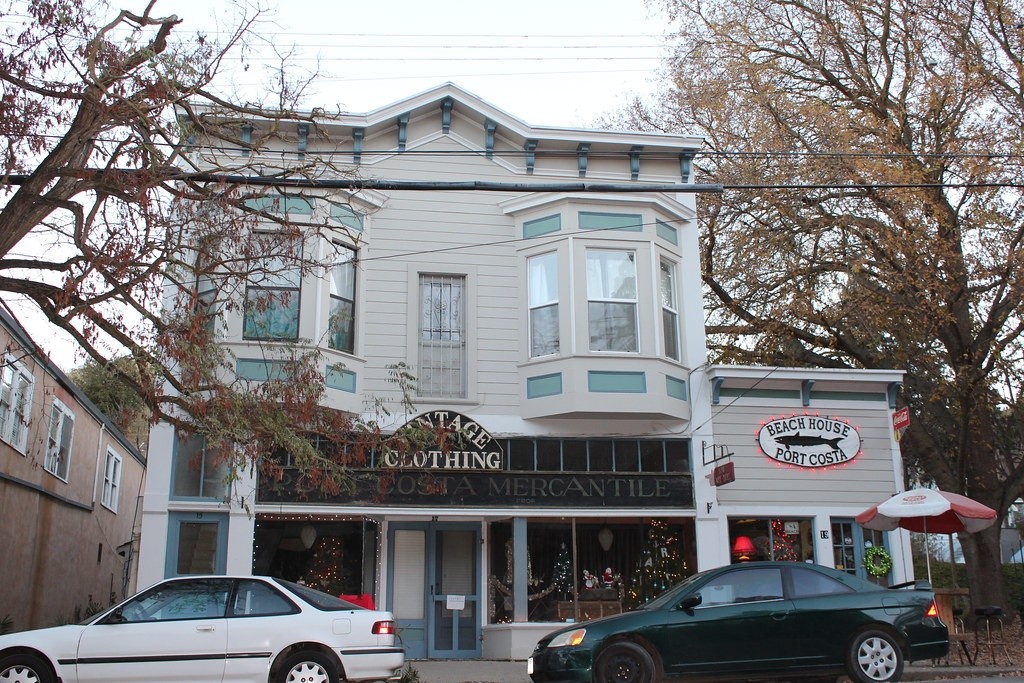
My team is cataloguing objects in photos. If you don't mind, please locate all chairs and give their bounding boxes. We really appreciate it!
[218,600,225,616]
[206,593,218,617]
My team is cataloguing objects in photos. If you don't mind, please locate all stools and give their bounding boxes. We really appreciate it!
[937,607,973,667]
[973,605,1013,666]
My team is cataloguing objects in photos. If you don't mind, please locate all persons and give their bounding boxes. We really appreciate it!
[583,570,598,589]
[602,568,613,587]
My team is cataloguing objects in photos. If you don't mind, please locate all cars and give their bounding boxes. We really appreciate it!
[0,576,407,683]
[527,560,951,683]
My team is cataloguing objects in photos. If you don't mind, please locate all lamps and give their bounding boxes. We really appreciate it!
[733,536,755,559]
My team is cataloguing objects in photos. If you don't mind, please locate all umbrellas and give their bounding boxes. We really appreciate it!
[855,487,997,585]
